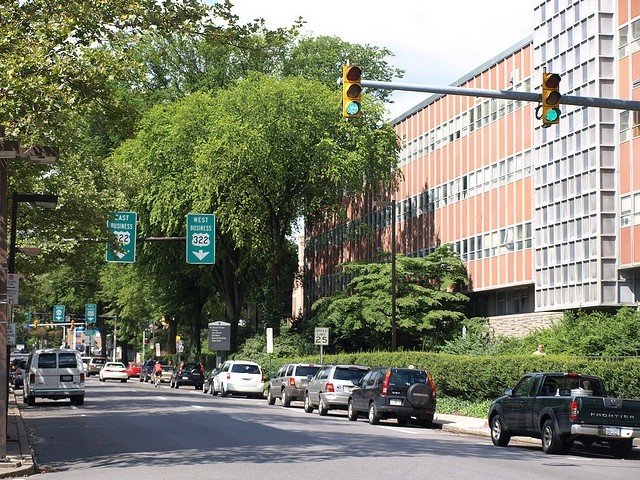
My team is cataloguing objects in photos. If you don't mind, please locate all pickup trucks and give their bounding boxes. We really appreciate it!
[488,372,640,454]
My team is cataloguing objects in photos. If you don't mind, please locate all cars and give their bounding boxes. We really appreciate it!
[99,362,128,382]
[150,365,174,384]
[127,362,144,380]
[203,368,220,394]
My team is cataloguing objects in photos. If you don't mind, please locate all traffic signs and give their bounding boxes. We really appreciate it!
[186,214,215,264]
[106,212,136,263]
[85,304,96,322]
[53,304,64,322]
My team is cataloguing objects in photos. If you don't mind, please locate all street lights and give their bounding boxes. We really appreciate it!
[6,191,58,438]
[374,200,397,351]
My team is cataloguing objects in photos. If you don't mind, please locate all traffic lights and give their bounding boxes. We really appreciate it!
[71,320,75,329]
[343,67,362,117]
[34,320,38,329]
[543,73,561,123]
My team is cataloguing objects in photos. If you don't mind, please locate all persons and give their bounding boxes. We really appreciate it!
[153,361,163,388]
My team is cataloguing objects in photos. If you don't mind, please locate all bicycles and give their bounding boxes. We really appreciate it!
[152,372,162,389]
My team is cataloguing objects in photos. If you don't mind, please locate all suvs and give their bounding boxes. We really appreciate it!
[348,367,436,425]
[86,357,108,377]
[304,365,371,415]
[10,358,22,385]
[15,360,28,389]
[267,364,321,406]
[170,361,205,389]
[140,358,158,382]
[23,348,85,405]
[212,359,264,399]
